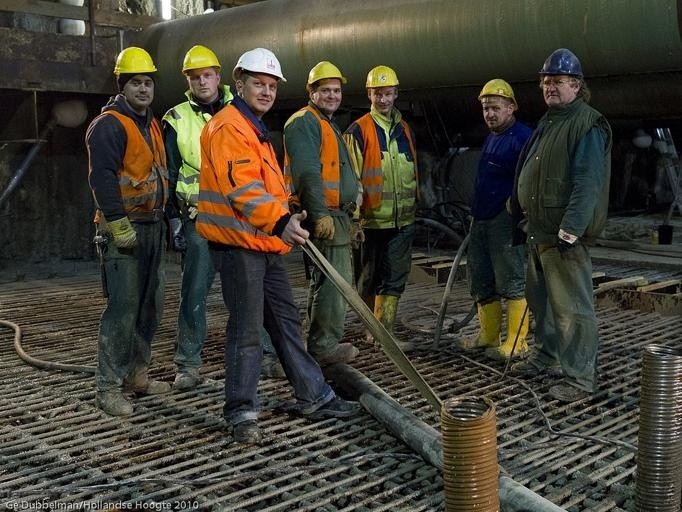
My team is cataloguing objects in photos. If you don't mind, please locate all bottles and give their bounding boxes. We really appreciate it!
[649,224,659,245]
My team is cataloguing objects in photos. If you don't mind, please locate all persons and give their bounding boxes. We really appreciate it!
[506,44,617,404]
[455,77,540,362]
[341,64,420,353]
[84,44,171,419]
[161,44,288,392]
[195,46,366,444]
[281,58,366,363]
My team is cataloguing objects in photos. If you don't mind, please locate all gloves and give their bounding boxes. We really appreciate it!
[312,215,335,240]
[168,217,186,253]
[351,219,367,251]
[106,215,138,250]
[556,237,575,259]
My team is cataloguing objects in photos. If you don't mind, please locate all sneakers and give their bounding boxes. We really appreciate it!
[313,342,360,368]
[261,352,287,379]
[94,388,134,417]
[233,420,263,445]
[123,375,170,394]
[172,367,201,389]
[301,393,362,419]
[548,382,590,403]
[510,363,563,377]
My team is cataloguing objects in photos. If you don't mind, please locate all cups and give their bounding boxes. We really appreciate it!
[658,225,674,245]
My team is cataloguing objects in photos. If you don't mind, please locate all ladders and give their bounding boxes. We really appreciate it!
[654,128,682,215]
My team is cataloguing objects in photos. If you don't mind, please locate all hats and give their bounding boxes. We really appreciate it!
[117,73,157,97]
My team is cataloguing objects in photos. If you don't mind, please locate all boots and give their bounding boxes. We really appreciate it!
[454,299,504,353]
[372,294,414,354]
[484,295,530,362]
[361,295,376,345]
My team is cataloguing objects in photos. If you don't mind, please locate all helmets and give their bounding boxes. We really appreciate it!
[113,46,157,75]
[477,78,519,112]
[232,47,287,83]
[365,65,400,88]
[538,47,584,77]
[181,44,221,76]
[305,59,347,94]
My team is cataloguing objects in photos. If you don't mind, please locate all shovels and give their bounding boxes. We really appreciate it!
[658,163,682,245]
